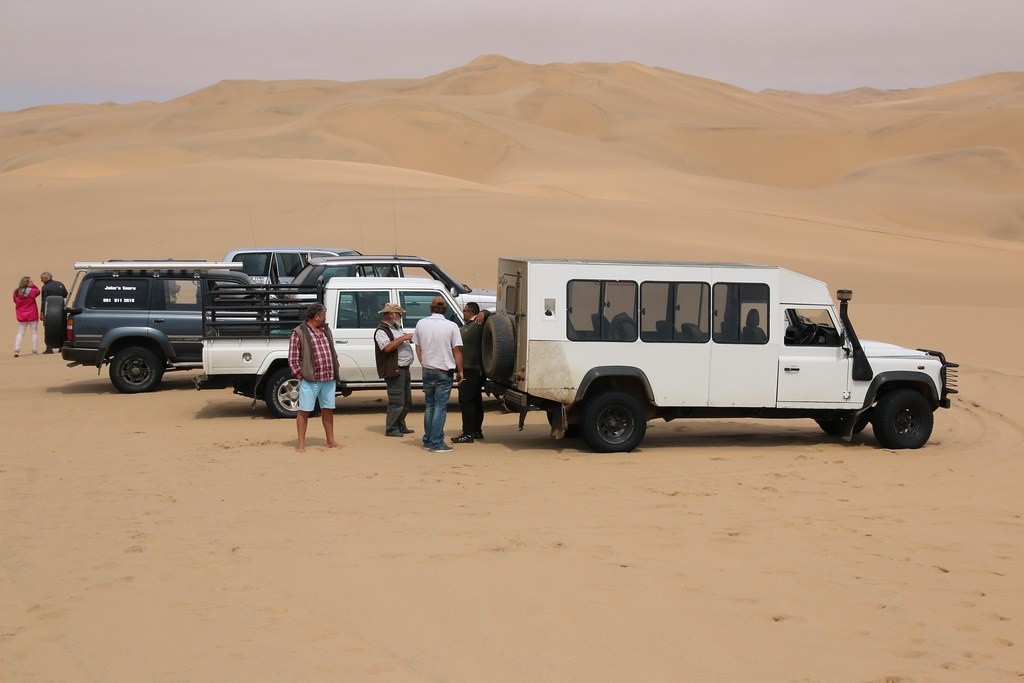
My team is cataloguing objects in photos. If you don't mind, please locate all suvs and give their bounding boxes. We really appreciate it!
[196,249,377,318]
[44,259,280,393]
[479,260,959,452]
[285,255,500,320]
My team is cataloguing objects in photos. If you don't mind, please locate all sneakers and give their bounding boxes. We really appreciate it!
[422,444,431,450]
[430,445,454,452]
[475,433,485,439]
[450,434,474,443]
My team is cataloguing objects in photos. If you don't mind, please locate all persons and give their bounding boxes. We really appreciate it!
[167,258,180,304]
[288,304,344,453]
[411,297,463,453]
[451,302,494,443]
[40,273,68,354]
[13,276,41,358]
[374,303,415,437]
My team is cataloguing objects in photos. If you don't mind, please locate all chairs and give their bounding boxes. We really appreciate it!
[721,304,743,342]
[591,314,611,338]
[608,312,637,341]
[743,309,766,344]
[681,323,705,342]
[656,320,679,340]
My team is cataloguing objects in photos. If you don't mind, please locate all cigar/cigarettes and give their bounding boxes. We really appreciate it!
[462,379,466,381]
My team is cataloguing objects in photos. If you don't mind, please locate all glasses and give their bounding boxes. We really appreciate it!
[463,309,467,312]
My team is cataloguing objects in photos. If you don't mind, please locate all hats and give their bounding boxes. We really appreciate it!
[377,303,407,314]
[431,297,447,307]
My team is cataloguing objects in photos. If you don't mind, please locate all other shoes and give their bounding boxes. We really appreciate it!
[58,349,61,353]
[32,350,39,356]
[43,349,53,354]
[14,350,20,357]
[386,432,403,438]
[402,427,415,433]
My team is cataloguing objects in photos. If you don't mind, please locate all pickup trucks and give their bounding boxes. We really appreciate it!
[194,276,467,419]
